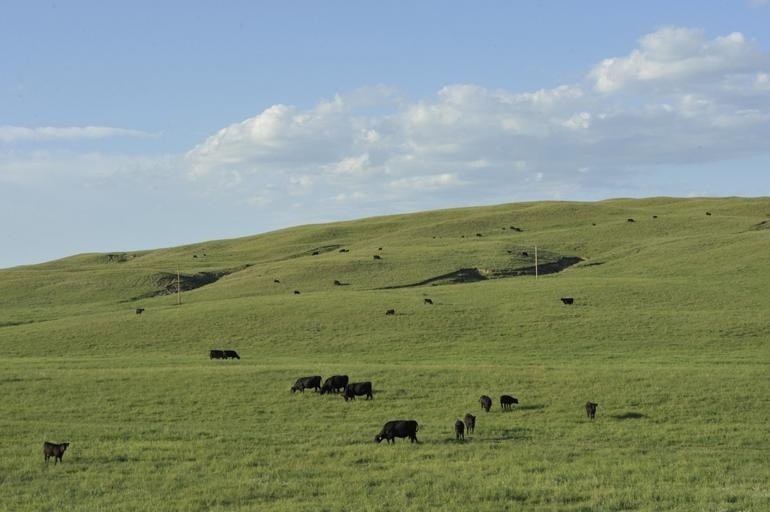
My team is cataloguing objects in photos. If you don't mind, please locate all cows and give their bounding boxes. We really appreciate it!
[561,297,574,305]
[210,350,240,360]
[454,414,476,442]
[43,441,70,465]
[479,395,491,412]
[290,374,374,402]
[585,401,598,419]
[375,420,419,445]
[500,395,519,410]
